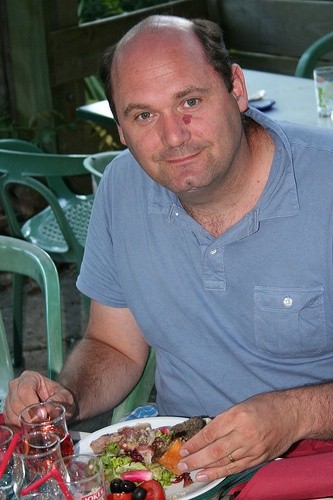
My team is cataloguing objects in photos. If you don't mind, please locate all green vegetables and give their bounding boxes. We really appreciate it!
[86,429,184,486]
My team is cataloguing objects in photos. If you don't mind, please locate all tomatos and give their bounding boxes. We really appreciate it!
[104,479,166,500]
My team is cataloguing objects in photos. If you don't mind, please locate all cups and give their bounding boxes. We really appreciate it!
[313,66,333,117]
[0,401,105,500]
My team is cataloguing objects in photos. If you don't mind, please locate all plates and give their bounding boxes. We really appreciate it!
[248,99,275,112]
[70,416,228,500]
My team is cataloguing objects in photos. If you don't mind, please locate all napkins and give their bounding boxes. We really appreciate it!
[228,438,333,500]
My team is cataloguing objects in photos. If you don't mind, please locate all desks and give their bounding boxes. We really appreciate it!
[77,68,332,141]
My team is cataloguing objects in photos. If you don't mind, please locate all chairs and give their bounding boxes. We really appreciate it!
[0,234,62,414]
[2,139,90,369]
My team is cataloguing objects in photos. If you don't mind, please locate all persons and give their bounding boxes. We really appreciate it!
[4,14,333,482]
